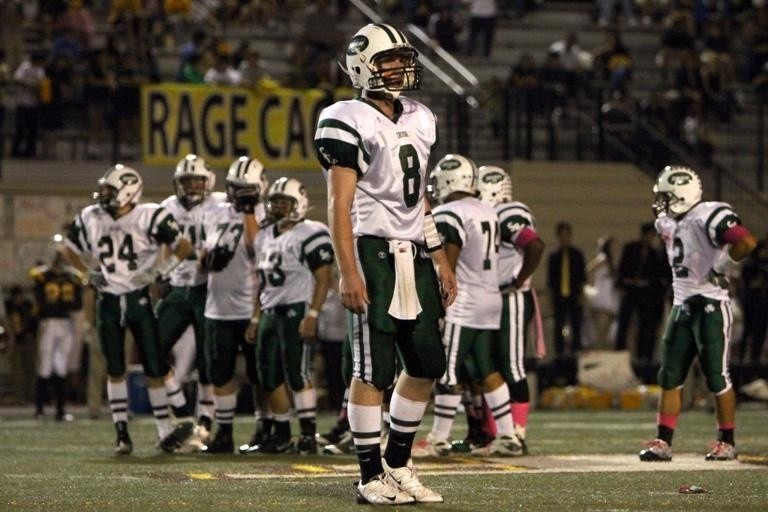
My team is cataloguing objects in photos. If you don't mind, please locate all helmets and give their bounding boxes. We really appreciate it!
[173,155,308,222]
[652,166,702,218]
[338,22,422,99]
[91,162,143,208]
[428,153,512,207]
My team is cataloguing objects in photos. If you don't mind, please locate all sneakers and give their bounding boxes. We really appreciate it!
[639,439,672,461]
[706,441,738,460]
[116,416,357,454]
[36,411,73,422]
[410,434,528,458]
[357,458,444,504]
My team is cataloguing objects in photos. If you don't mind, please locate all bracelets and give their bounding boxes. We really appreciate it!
[250,316,260,325]
[308,308,320,319]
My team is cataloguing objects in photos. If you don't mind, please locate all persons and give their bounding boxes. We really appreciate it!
[1,1,362,162]
[195,154,268,460]
[545,215,768,370]
[311,21,464,508]
[151,150,227,449]
[0,238,134,425]
[377,1,768,170]
[638,161,759,462]
[61,160,196,456]
[244,175,335,458]
[316,151,546,461]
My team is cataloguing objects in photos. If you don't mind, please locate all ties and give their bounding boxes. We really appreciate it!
[561,252,570,296]
